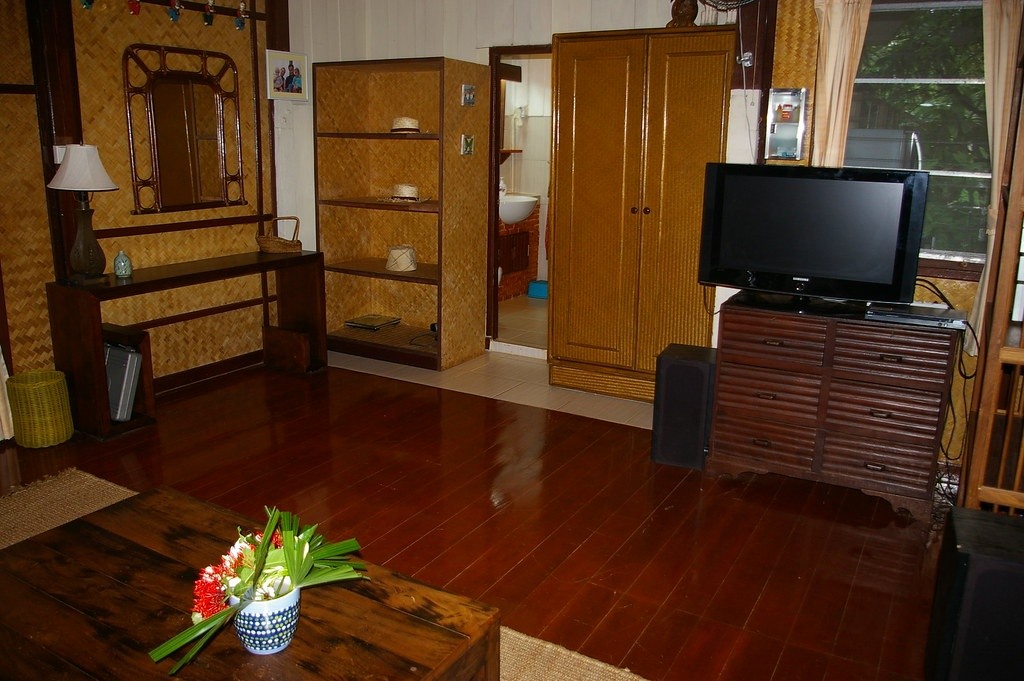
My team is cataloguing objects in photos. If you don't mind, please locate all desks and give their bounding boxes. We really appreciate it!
[0,483,502,681]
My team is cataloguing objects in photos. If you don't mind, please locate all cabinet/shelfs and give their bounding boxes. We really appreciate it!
[705,290,969,523]
[312,56,492,373]
[547,26,735,401]
[45,249,328,437]
[959,10,1023,519]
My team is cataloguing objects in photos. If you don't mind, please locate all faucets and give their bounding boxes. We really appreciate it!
[500,176,507,193]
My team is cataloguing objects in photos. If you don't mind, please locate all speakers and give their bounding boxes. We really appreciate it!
[649,342,717,469]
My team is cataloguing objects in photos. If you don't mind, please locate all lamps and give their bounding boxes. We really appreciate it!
[46,140,121,284]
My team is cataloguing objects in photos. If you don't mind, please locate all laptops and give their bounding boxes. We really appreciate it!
[345,315,402,331]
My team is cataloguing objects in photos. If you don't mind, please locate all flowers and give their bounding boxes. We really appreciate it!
[147,504,370,675]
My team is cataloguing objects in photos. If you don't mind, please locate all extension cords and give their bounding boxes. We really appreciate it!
[910,301,949,310]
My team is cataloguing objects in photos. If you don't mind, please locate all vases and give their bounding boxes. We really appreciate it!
[228,587,301,656]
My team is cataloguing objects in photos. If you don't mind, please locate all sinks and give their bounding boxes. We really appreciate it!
[499,193,539,224]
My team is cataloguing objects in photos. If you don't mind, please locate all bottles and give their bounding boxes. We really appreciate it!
[114,250,132,278]
[775,103,798,122]
[499,176,507,196]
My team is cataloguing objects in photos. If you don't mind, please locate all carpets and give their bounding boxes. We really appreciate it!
[0,465,650,681]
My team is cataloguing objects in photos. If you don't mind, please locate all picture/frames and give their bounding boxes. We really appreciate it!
[267,49,308,102]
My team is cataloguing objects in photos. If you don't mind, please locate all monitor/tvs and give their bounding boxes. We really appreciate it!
[696,163,929,317]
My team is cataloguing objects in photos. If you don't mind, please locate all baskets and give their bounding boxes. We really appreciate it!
[256,216,302,254]
